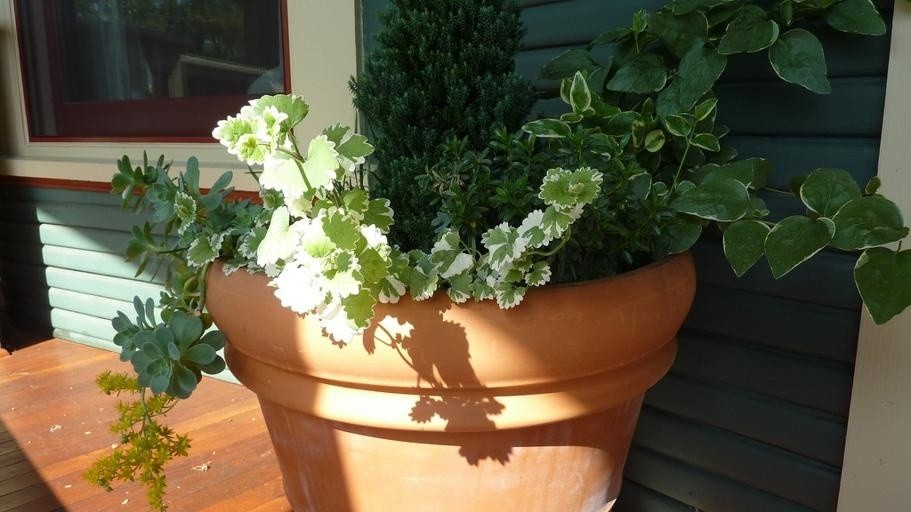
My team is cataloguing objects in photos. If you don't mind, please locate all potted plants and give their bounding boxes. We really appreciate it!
[89,1,911,512]
